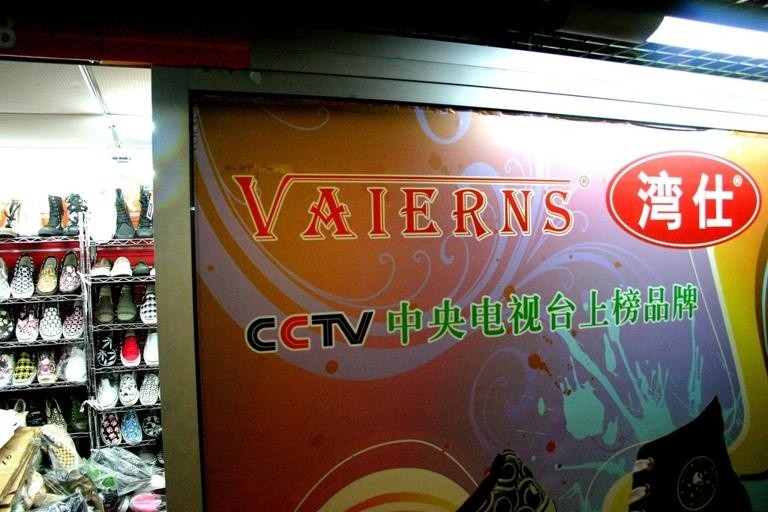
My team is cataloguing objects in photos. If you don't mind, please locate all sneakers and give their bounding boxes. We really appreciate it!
[62,296,88,341]
[95,283,115,326]
[111,256,133,277]
[132,259,150,276]
[15,303,41,345]
[36,255,59,297]
[96,370,162,448]
[0,306,15,342]
[39,299,64,343]
[58,249,82,294]
[1,345,88,389]
[116,284,138,323]
[96,330,118,368]
[10,253,35,299]
[1,390,90,434]
[143,328,161,368]
[119,328,142,370]
[0,257,11,303]
[139,284,158,327]
[90,257,112,277]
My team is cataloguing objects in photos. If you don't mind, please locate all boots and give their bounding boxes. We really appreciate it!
[135,183,155,239]
[109,187,136,239]
[38,195,64,236]
[0,198,21,238]
[61,192,87,236]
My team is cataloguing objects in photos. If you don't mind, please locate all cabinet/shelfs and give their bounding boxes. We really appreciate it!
[84,210,163,449]
[0,212,94,457]
